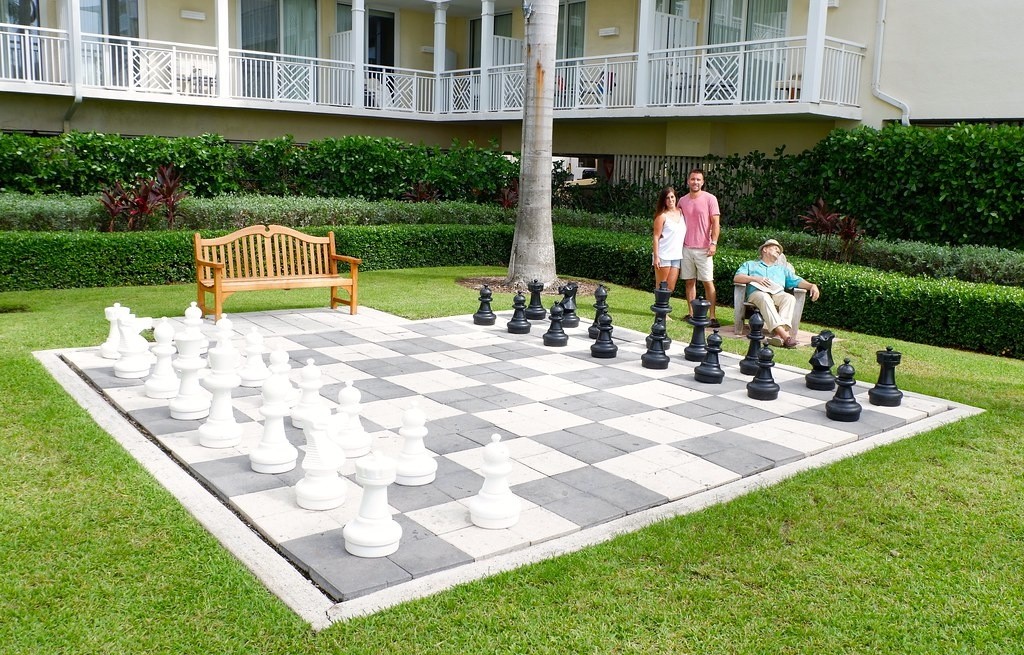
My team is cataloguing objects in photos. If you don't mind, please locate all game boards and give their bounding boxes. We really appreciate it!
[32,309,987,631]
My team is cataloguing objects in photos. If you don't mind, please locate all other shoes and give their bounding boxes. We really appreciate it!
[681,315,692,321]
[710,319,720,327]
[766,336,783,346]
[785,336,800,348]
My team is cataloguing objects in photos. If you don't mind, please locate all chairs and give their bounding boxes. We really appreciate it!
[667,64,735,99]
[733,253,807,341]
[580,71,616,107]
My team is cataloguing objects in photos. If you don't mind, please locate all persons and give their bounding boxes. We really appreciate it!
[733,239,820,347]
[652,186,712,320]
[659,168,720,328]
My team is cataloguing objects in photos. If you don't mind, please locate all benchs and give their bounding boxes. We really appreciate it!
[194,224,361,324]
[169,61,217,93]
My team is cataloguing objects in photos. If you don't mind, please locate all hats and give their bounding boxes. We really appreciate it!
[759,239,784,255]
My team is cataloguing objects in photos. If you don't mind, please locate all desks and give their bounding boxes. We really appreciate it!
[775,79,802,99]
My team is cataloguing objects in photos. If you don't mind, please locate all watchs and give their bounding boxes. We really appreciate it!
[710,240,717,245]
